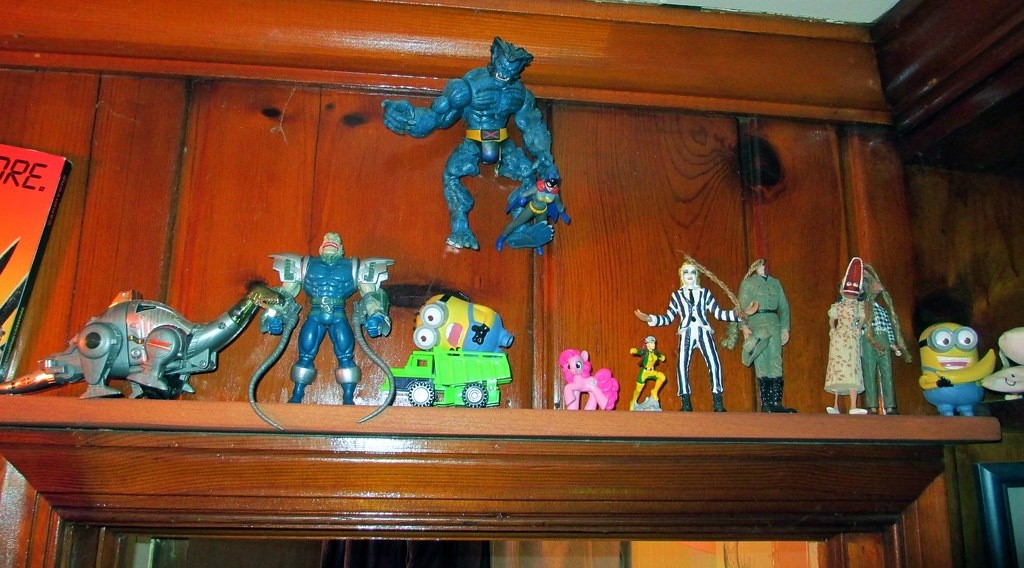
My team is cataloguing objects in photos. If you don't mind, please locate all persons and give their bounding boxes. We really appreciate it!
[824,257,913,415]
[248,231,394,430]
[381,36,559,250]
[629,259,798,414]
[496,173,571,256]
